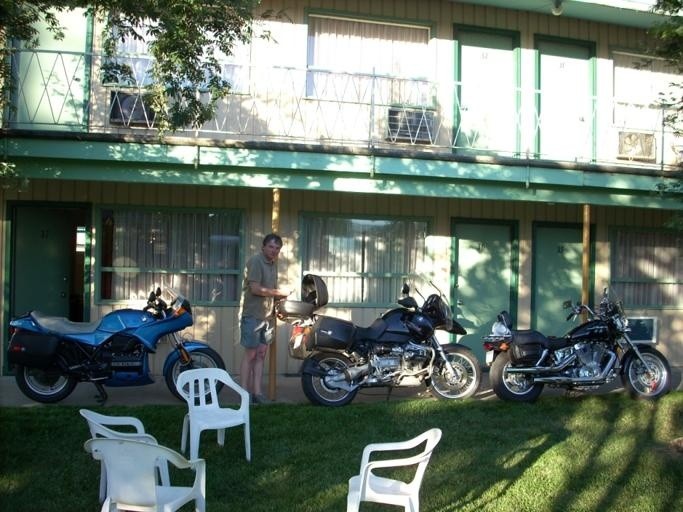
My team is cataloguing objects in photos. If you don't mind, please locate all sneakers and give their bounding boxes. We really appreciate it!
[251,393,271,405]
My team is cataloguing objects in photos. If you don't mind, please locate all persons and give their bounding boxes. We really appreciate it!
[238,234,289,406]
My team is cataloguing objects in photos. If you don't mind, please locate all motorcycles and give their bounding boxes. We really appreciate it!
[271,269,481,405]
[5,280,226,405]
[482,281,672,404]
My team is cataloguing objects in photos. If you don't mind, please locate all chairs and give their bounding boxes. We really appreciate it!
[175,368,251,470]
[345,428,442,512]
[78,409,207,512]
